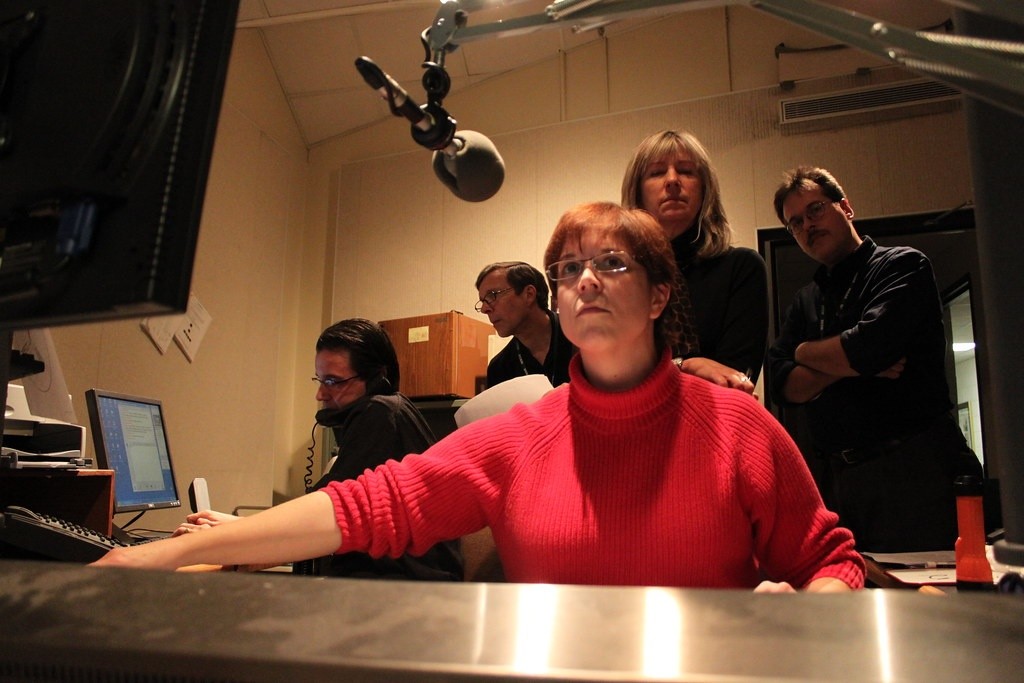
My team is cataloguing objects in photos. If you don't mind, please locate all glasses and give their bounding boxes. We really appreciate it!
[786,198,834,235]
[545,251,644,281]
[476,286,516,312]
[311,374,361,387]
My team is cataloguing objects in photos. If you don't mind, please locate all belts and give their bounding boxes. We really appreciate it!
[823,422,934,465]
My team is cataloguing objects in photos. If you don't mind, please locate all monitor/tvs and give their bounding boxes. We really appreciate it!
[85,389,181,545]
[0,0,240,469]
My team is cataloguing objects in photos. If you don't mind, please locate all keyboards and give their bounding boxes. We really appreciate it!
[129,535,173,547]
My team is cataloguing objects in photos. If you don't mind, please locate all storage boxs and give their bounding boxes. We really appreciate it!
[377,310,496,399]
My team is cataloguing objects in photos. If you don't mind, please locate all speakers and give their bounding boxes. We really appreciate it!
[189,478,211,513]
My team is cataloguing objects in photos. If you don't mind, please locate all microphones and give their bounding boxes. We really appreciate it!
[356,56,506,203]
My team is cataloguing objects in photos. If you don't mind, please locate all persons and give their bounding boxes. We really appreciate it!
[172,318,464,583]
[761,167,984,553]
[621,129,769,400]
[88,201,869,592]
[475,261,574,388]
